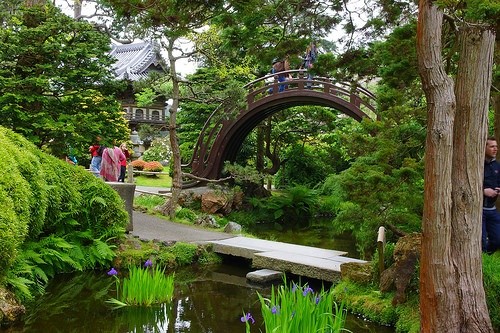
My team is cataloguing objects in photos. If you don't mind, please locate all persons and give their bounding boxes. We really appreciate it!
[88,135,102,177]
[481,137,500,255]
[100,139,125,182]
[267,41,317,95]
[118,143,129,182]
[66,155,77,166]
